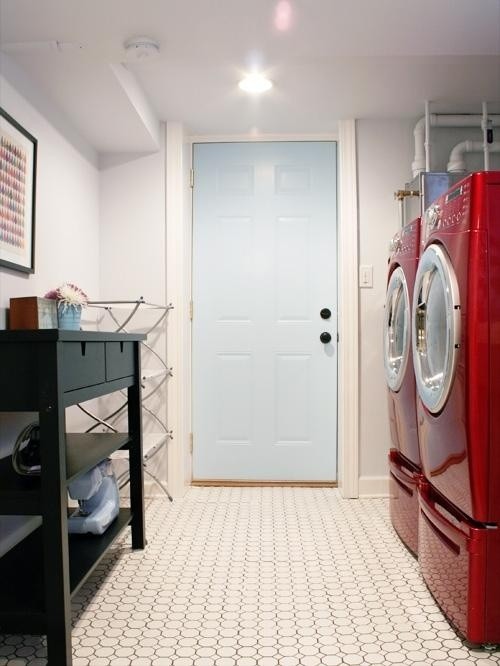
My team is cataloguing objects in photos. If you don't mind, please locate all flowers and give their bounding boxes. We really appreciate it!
[44,281,88,314]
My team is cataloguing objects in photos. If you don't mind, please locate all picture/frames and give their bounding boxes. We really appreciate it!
[0,107,38,274]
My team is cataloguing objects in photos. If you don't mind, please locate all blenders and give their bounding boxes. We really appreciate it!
[67,459,120,535]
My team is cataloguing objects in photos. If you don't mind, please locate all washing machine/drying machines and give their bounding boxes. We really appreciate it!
[410,172,500,648]
[380,218,421,561]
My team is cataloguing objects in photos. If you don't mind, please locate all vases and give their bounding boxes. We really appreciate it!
[57,303,82,330]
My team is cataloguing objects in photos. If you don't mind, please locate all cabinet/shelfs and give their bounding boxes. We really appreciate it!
[87,298,174,503]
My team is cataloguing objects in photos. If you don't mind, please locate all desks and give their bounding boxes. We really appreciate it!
[0,331,147,666]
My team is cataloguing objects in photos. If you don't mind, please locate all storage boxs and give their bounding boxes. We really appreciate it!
[10,296,58,330]
